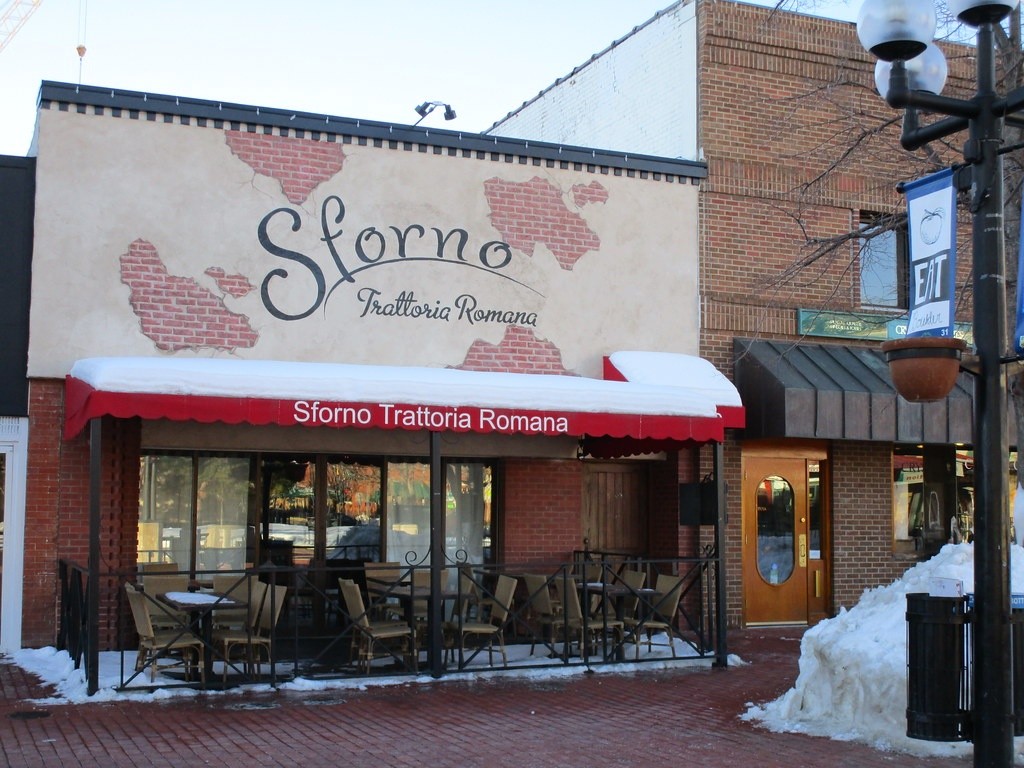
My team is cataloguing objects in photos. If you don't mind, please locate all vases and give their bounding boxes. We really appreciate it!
[879,337,968,403]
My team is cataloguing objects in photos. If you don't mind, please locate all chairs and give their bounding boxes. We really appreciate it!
[125,561,687,686]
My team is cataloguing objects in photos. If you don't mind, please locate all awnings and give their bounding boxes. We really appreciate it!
[730,342,1019,450]
[65,350,745,441]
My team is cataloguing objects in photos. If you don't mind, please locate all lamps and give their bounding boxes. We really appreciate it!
[413,101,456,129]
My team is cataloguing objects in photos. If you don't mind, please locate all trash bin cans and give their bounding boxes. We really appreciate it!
[893,577,1018,754]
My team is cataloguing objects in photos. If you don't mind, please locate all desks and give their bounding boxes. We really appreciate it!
[575,582,662,662]
[190,580,214,588]
[474,569,596,645]
[367,575,411,620]
[155,591,248,685]
[367,587,475,670]
